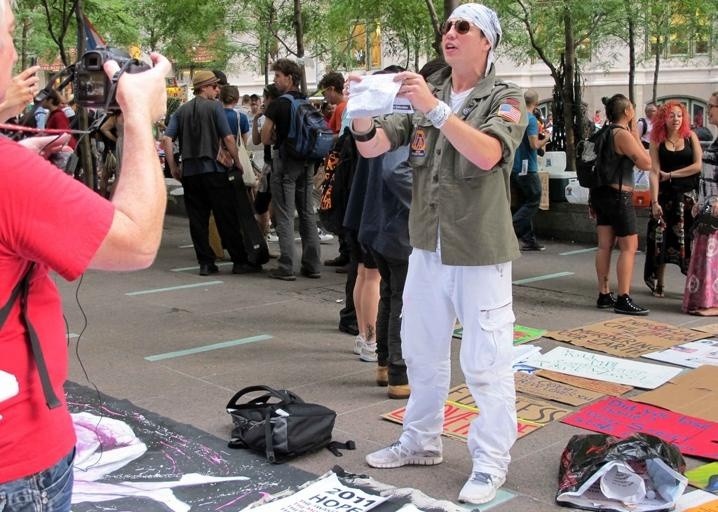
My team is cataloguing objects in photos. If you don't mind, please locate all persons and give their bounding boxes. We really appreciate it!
[22,87,125,200]
[509,91,718,314]
[1,63,42,126]
[162,59,449,399]
[342,3,525,506]
[1,3,169,511]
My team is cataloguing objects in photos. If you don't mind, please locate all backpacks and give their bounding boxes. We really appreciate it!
[21,108,46,137]
[225,384,355,466]
[279,94,336,174]
[574,123,628,207]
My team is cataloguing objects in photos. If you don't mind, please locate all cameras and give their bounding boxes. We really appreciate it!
[75,48,151,107]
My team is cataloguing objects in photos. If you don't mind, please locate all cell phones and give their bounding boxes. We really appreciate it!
[31,56,37,87]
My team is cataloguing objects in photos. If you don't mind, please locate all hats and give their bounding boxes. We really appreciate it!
[33,90,49,102]
[191,70,221,91]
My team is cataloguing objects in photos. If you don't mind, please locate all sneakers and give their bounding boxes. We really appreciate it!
[365,439,443,470]
[459,471,506,505]
[521,242,547,252]
[596,291,650,315]
[339,318,411,400]
[200,226,352,281]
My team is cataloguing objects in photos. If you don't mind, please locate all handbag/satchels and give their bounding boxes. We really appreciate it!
[235,141,257,187]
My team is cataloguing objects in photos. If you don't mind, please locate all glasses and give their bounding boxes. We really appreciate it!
[208,84,218,90]
[440,19,471,34]
